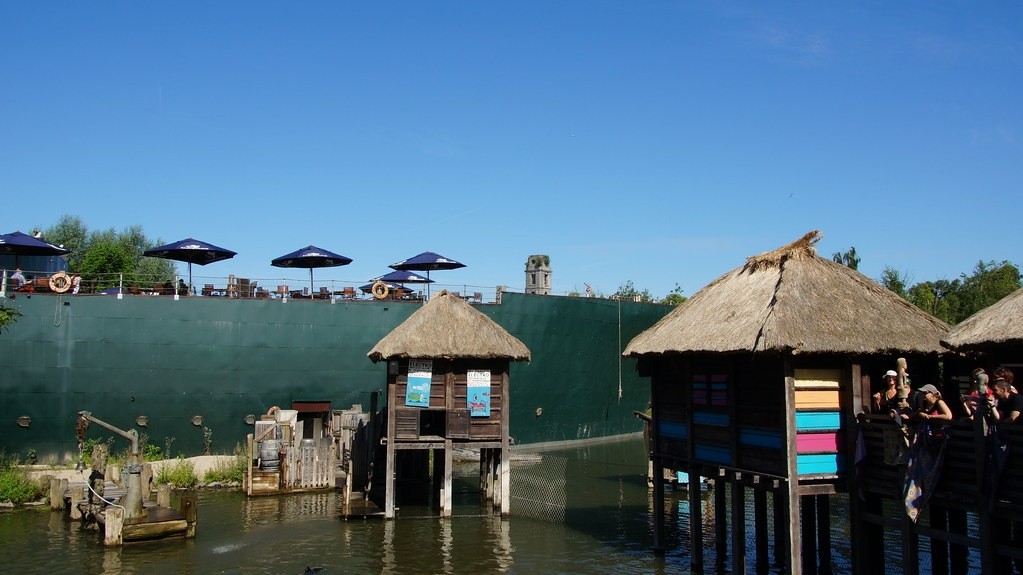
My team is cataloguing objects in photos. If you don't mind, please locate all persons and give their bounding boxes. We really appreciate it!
[10,266,35,293]
[67,272,82,293]
[872,364,1023,504]
[177,279,187,295]
[163,280,175,294]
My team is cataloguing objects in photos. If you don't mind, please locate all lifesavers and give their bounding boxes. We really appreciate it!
[48,273,72,293]
[371,281,390,298]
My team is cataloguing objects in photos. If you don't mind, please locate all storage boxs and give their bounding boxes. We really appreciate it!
[255,409,298,442]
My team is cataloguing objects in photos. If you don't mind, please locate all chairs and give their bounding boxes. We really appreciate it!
[7,278,19,292]
[277,286,290,296]
[205,284,213,297]
[155,284,168,295]
[344,285,355,300]
[127,283,140,294]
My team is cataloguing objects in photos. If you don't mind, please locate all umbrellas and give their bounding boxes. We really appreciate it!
[270,245,353,300]
[388,251,468,300]
[140,238,238,296]
[360,282,413,295]
[370,270,435,299]
[0,232,69,270]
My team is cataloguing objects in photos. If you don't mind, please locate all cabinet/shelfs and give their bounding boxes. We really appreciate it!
[237,278,251,297]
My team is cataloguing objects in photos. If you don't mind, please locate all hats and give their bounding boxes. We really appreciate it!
[918,384,937,394]
[882,370,897,378]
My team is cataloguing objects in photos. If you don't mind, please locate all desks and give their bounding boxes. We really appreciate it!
[212,289,227,298]
